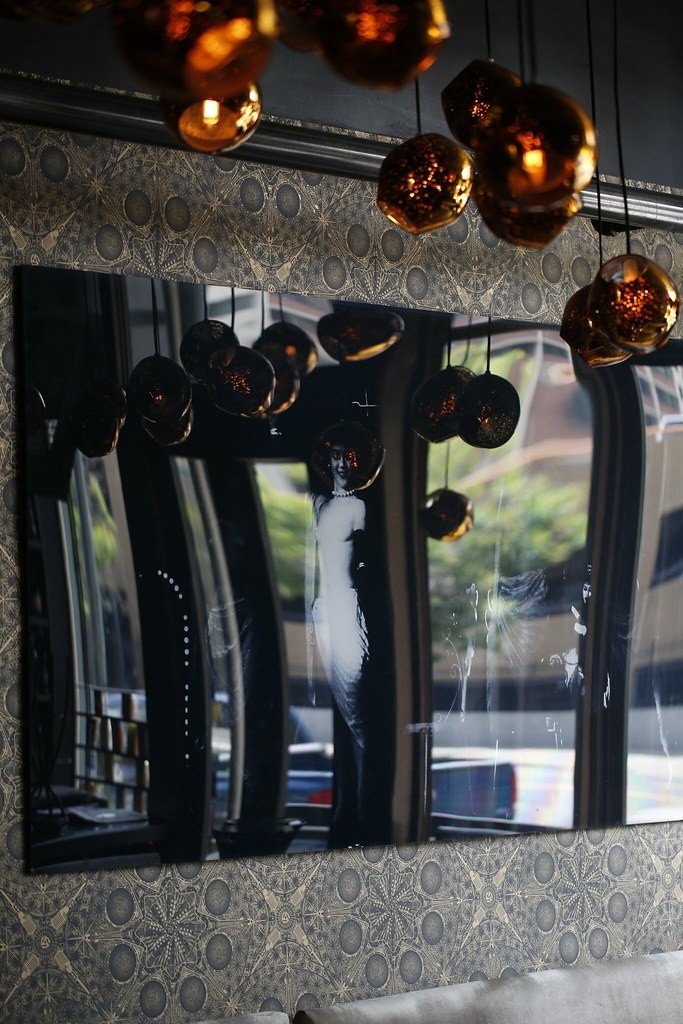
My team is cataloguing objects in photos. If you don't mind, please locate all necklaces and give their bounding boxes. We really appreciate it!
[331,489,355,497]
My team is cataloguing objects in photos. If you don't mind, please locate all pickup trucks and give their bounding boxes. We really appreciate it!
[85,692,516,854]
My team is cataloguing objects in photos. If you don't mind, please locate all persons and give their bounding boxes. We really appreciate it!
[549,560,612,707]
[304,439,377,827]
